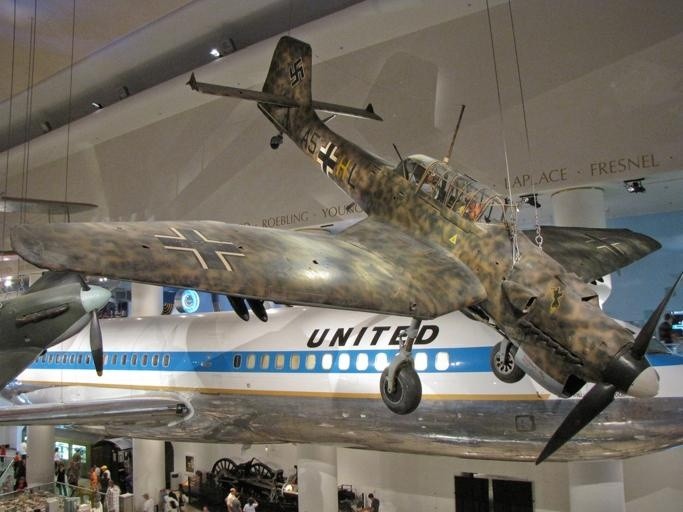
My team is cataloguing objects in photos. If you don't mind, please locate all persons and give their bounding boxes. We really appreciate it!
[658,312,675,352]
[225,488,258,512]
[368,493,379,512]
[0,445,27,492]
[142,483,184,512]
[54,446,133,512]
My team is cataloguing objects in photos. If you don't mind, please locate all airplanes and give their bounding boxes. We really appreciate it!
[0,197,115,393]
[12,36,683,466]
[0,305,683,465]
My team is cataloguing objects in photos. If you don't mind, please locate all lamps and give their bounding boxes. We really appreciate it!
[209,46,222,58]
[40,121,51,133]
[119,86,129,97]
[92,102,103,109]
[220,38,236,54]
